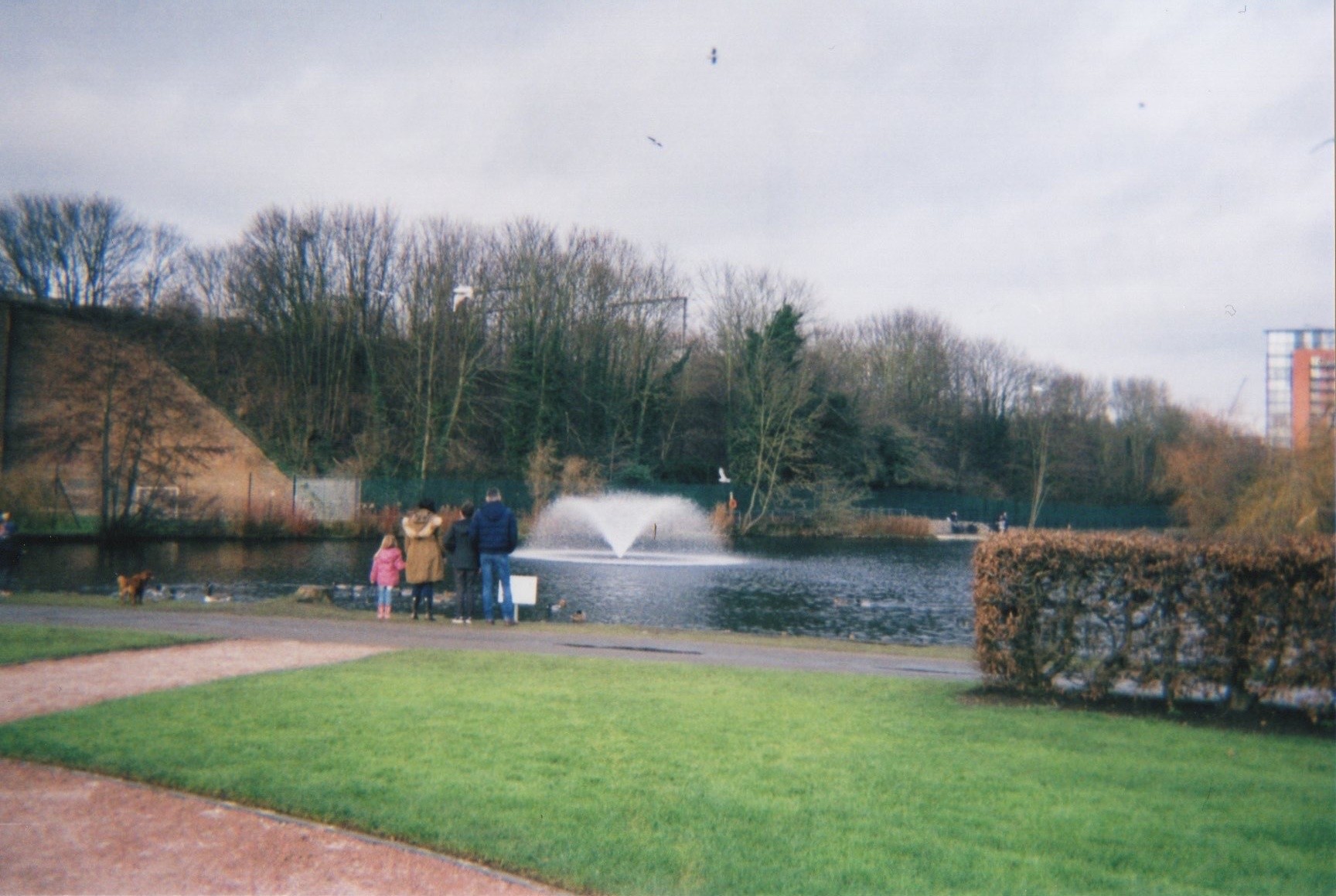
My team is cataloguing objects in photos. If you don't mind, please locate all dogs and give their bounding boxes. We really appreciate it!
[116,571,155,610]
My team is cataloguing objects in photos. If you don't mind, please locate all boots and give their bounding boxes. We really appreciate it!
[409,597,419,620]
[384,605,392,619]
[424,596,434,621]
[377,606,384,619]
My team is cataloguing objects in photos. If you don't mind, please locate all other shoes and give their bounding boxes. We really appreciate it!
[504,619,518,626]
[466,616,472,624]
[486,619,495,626]
[452,616,464,623]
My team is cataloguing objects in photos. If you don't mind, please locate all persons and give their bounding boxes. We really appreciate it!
[995,512,1008,533]
[470,486,520,627]
[945,511,958,523]
[0,511,17,540]
[548,599,587,621]
[335,583,459,604]
[145,580,232,604]
[399,499,445,621]
[443,498,482,623]
[370,534,406,619]
